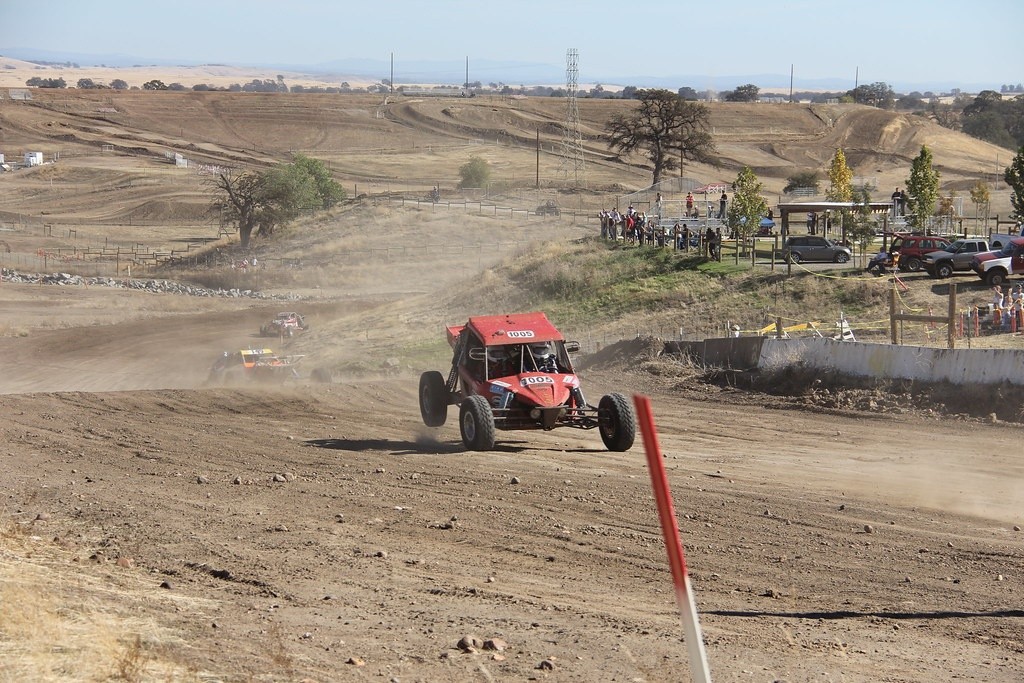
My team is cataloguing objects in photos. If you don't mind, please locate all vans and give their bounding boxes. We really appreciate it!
[888,234,952,272]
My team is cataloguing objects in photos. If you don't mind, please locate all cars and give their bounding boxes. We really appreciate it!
[419,312,637,452]
[263,312,307,337]
[209,349,332,384]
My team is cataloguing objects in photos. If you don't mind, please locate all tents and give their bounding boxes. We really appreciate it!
[736,213,776,227]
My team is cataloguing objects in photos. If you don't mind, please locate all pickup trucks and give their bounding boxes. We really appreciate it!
[920,239,1002,278]
[968,224,1024,285]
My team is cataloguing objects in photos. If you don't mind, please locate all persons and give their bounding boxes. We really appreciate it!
[992,284,1024,329]
[806,211,833,234]
[598,190,728,261]
[476,341,566,382]
[891,187,906,216]
[864,247,900,278]
[767,206,774,234]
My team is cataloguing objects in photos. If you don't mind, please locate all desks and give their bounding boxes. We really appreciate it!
[884,267,901,276]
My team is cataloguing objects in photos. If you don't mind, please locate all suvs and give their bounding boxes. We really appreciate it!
[781,236,852,264]
